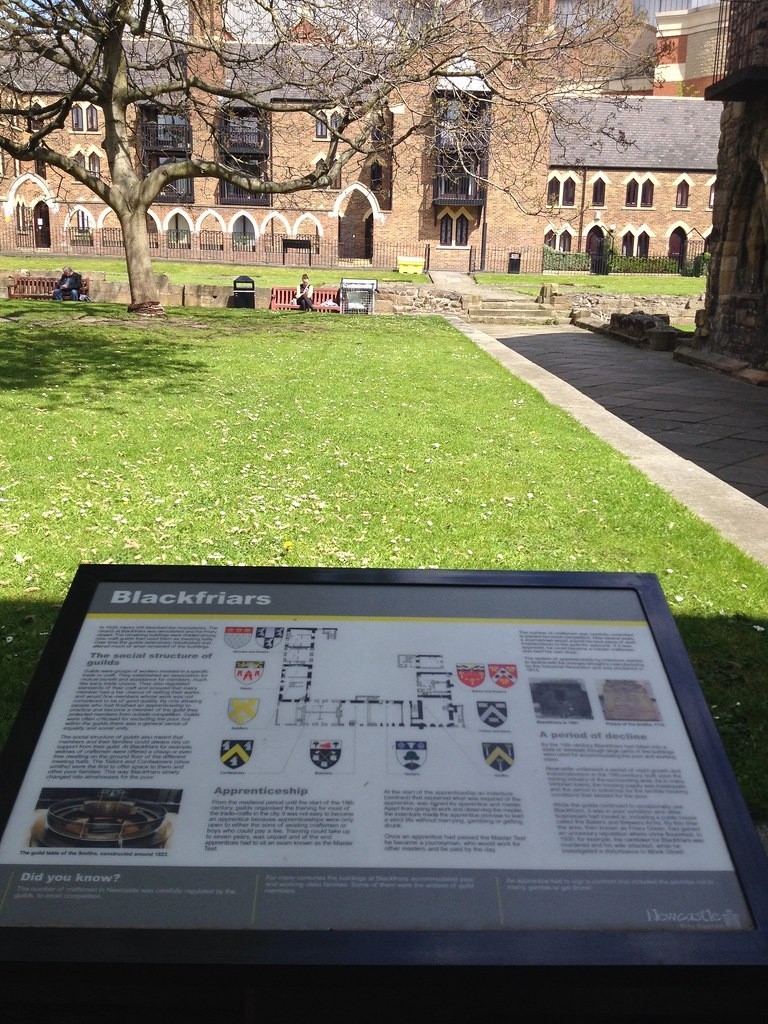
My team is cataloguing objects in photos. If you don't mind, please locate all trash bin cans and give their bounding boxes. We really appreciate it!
[233,276,255,309]
[507,252,521,274]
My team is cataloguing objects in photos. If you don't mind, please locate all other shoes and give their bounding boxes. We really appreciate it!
[310,304,314,309]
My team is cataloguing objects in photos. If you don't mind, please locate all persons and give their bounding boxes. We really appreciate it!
[52,266,81,302]
[295,273,315,311]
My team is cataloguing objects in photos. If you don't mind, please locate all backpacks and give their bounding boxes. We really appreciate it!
[79,294,94,302]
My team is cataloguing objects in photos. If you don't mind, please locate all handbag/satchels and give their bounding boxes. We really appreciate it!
[291,298,297,305]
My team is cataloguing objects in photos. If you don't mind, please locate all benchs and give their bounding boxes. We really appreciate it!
[6,276,90,301]
[268,286,340,313]
